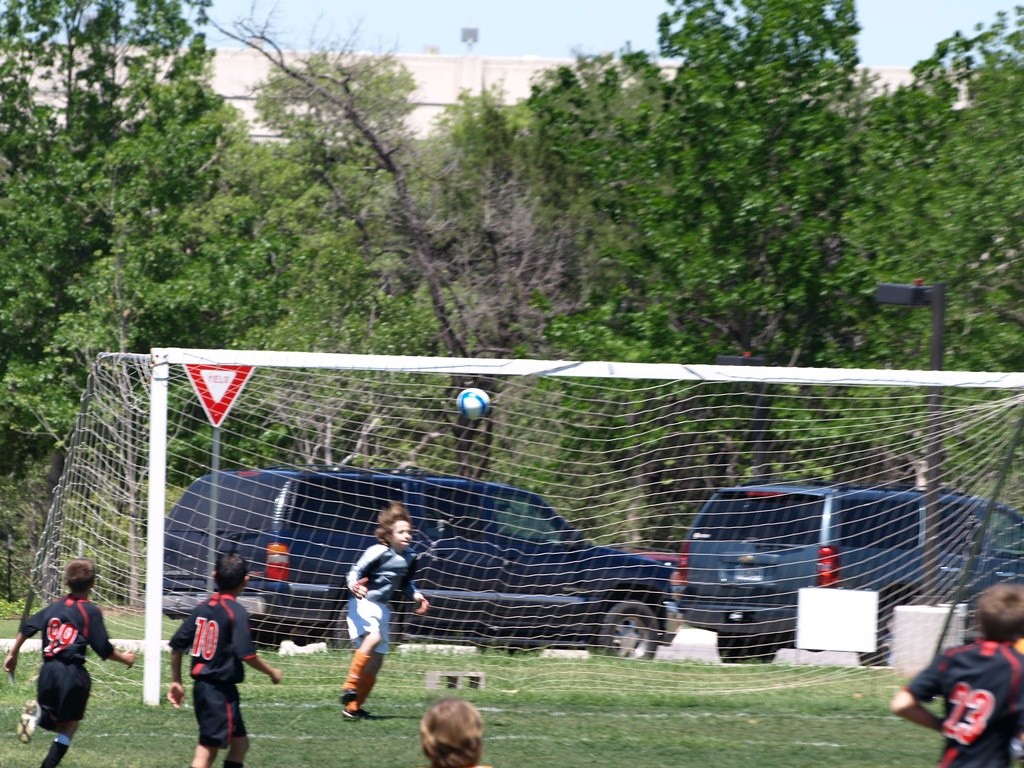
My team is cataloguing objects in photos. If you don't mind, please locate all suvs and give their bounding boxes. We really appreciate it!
[663,481,1024,666]
[162,464,681,660]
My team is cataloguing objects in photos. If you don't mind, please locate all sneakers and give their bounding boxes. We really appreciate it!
[341,708,375,719]
[339,688,358,705]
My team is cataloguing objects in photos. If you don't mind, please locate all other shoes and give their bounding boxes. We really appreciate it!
[16,700,42,744]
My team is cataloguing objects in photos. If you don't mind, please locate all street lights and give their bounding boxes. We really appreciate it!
[870,279,944,605]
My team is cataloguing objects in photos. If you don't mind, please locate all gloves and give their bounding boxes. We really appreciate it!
[413,593,430,615]
[346,578,368,600]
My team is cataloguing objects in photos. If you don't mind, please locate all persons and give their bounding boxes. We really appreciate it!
[3,560,134,768]
[890,583,1024,768]
[338,502,429,718]
[418,698,493,768]
[167,558,281,768]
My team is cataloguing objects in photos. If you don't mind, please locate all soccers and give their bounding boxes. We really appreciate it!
[456,388,492,420]
